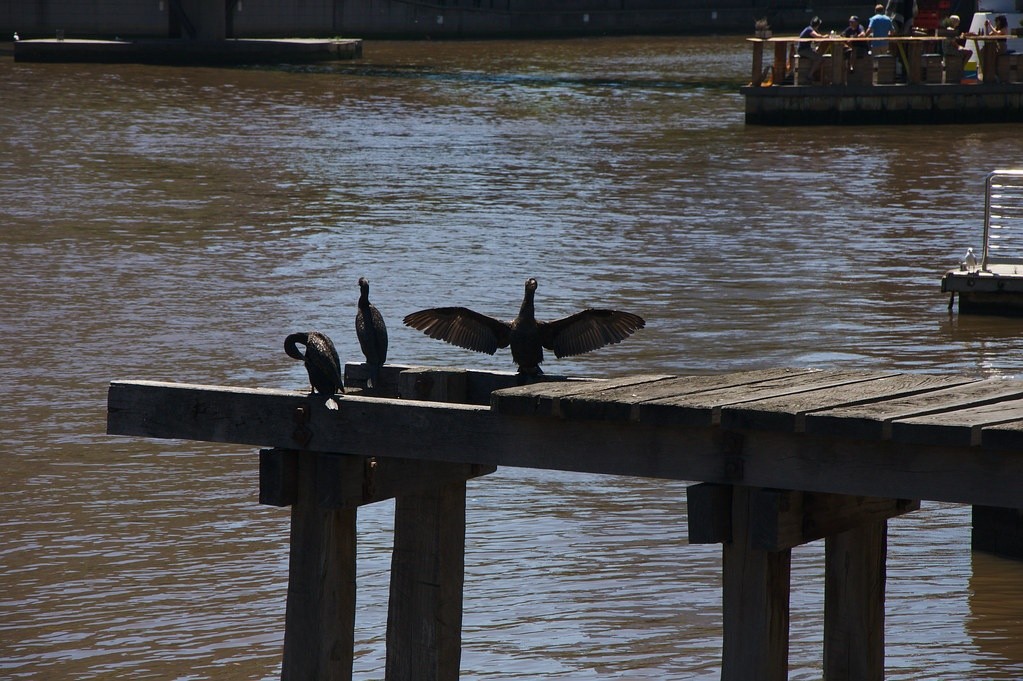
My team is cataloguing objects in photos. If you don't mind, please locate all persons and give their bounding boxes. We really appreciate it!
[839,14,865,59]
[885,0,921,36]
[984,14,1008,55]
[942,14,973,69]
[864,4,894,56]
[796,16,830,83]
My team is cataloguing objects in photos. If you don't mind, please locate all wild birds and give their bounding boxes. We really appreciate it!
[284,331,345,400]
[403,278,645,375]
[354,276,389,366]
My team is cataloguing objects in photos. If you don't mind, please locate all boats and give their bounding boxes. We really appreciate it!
[736,0,1023,123]
[940,169,1023,318]
